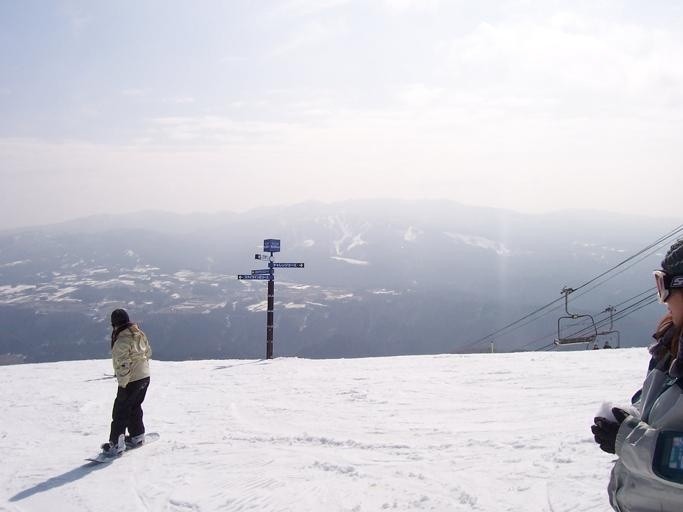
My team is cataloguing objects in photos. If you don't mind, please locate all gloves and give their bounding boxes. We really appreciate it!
[591,407,630,453]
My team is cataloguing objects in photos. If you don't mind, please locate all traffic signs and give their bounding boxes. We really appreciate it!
[267,262,304,269]
[238,275,274,280]
[254,254,271,262]
[250,269,274,275]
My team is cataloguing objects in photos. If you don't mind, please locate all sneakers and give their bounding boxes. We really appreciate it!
[127,434,142,444]
[102,443,121,454]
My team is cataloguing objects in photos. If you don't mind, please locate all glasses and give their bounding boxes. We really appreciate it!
[653,270,682,302]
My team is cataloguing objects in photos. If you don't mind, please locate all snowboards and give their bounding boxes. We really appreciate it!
[85,432,161,463]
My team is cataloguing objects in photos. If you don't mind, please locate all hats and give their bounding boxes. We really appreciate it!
[660,240,683,270]
[111,309,129,327]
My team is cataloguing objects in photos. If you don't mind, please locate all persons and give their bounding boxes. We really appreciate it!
[588,235,683,510]
[100,309,150,457]
